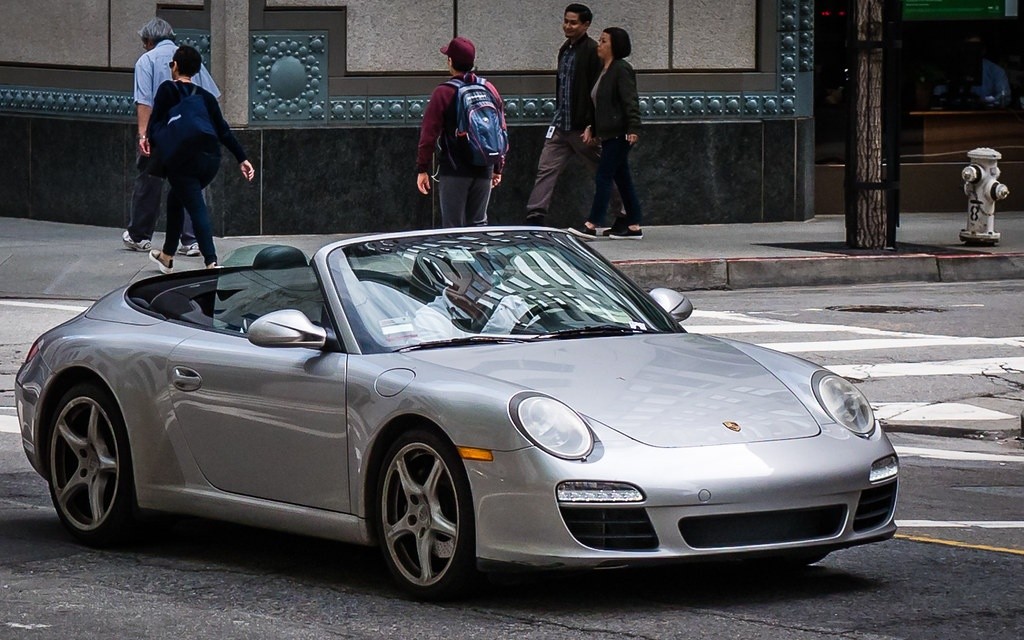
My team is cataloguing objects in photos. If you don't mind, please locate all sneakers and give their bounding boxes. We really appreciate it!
[609,227,643,239]
[149,249,173,273]
[568,225,597,240]
[123,231,152,251]
[177,242,201,256]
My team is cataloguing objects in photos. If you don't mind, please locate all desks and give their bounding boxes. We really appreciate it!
[902,110,1024,156]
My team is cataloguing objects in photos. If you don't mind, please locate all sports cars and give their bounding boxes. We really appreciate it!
[14,225,903,605]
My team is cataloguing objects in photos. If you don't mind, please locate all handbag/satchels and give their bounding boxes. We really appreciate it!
[148,81,217,180]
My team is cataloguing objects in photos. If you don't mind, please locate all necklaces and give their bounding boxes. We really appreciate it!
[177,77,191,81]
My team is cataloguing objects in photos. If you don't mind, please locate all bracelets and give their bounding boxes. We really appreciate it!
[136,135,147,139]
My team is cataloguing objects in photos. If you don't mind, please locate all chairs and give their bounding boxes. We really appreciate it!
[226,245,329,327]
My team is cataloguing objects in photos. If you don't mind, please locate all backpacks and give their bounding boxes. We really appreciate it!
[439,77,509,171]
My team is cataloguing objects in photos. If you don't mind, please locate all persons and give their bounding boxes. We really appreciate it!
[413,245,547,342]
[520,3,644,240]
[931,38,1011,107]
[122,17,255,275]
[417,37,509,229]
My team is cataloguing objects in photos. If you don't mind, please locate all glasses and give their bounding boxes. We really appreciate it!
[169,62,174,69]
[142,43,147,49]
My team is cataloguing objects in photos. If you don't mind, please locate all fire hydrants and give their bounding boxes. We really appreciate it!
[959,147,1010,246]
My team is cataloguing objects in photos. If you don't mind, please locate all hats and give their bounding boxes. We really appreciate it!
[440,37,476,64]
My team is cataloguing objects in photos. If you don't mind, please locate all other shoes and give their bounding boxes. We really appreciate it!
[603,215,628,236]
